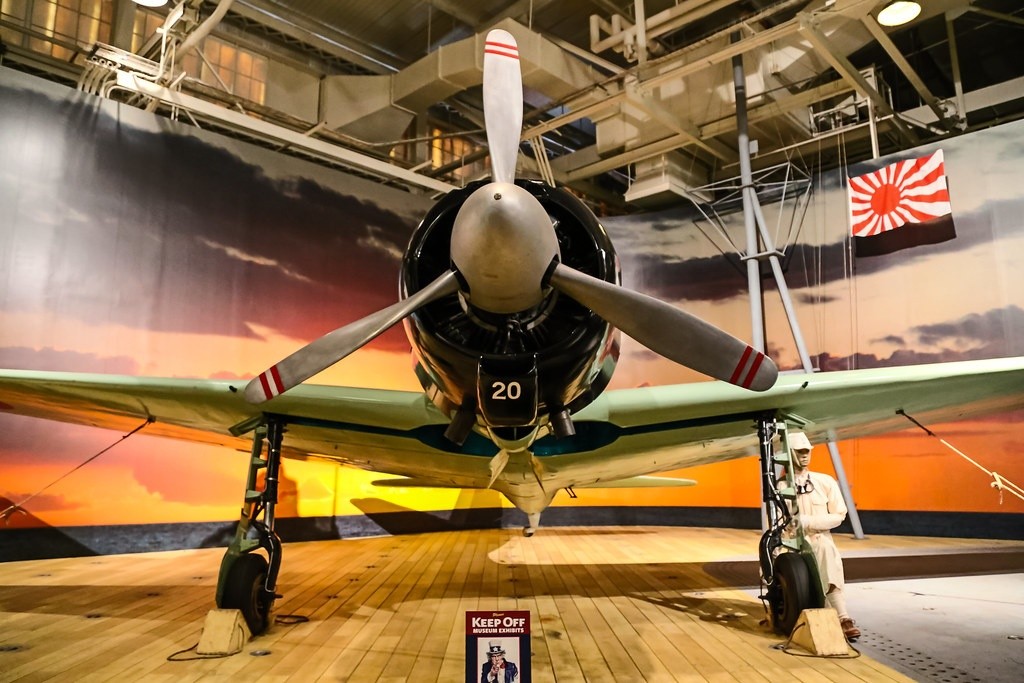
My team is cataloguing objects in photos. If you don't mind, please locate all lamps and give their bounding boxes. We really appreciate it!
[876,0,922,27]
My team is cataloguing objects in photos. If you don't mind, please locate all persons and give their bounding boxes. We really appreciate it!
[763,433,862,638]
[481,641,518,683]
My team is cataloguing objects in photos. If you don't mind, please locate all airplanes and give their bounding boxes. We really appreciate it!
[1,28,1024,637]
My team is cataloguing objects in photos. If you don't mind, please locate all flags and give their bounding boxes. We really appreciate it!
[845,147,954,237]
